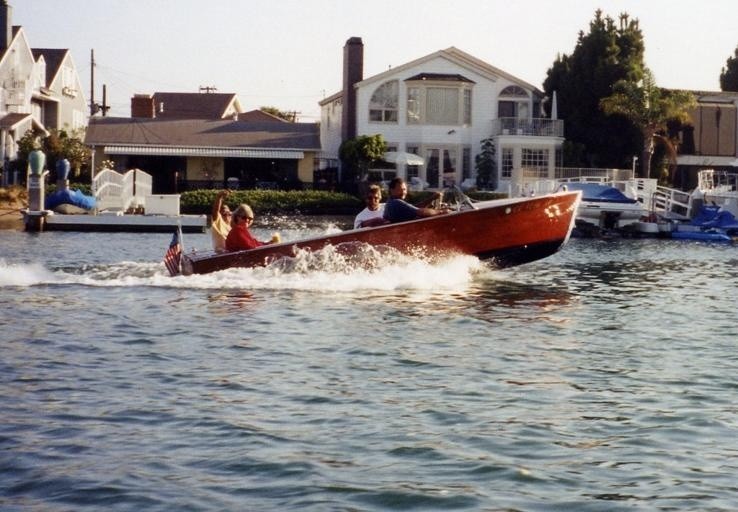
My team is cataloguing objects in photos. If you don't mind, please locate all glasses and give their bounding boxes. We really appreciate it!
[238,214,255,221]
[369,196,381,200]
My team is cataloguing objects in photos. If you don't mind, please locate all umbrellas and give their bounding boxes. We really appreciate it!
[382,151,426,177]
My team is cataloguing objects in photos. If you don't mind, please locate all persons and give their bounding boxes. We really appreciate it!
[211,189,276,249]
[354,176,452,229]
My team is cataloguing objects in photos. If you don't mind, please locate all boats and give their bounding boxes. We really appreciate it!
[179,189,584,279]
[553,169,738,242]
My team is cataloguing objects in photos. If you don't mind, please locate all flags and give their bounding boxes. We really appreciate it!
[163,222,182,276]
